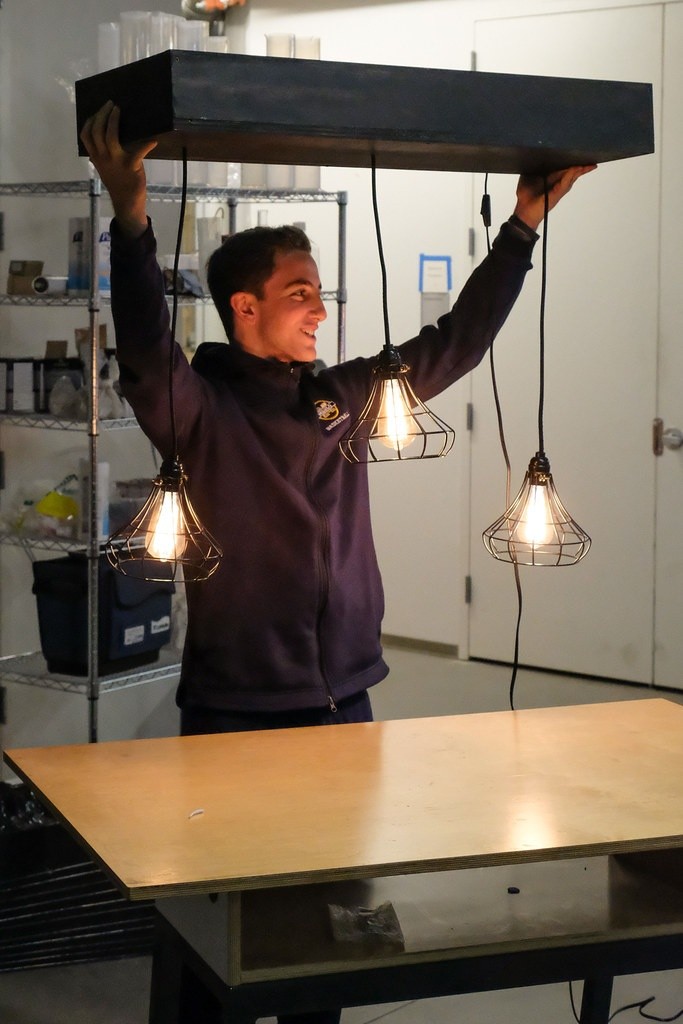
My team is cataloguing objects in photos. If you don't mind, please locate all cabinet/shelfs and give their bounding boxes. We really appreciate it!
[0,179,348,743]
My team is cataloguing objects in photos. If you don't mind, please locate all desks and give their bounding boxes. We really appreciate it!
[4,696,683,1024]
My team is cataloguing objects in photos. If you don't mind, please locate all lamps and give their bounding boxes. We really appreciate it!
[105,159,224,584]
[336,168,456,464]
[482,176,592,567]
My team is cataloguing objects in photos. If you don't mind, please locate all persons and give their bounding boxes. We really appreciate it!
[78,99,600,1023]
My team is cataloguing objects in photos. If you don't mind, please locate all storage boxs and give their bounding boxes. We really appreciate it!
[68,217,112,299]
[74,323,120,385]
[44,340,83,413]
[7,261,45,295]
